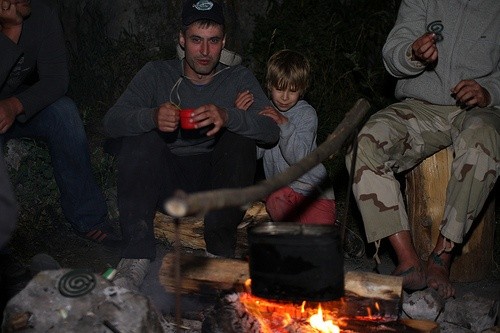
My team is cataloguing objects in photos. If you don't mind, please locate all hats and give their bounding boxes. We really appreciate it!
[182,0,226,27]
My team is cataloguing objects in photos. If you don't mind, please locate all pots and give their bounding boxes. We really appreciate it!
[247,223,345,300]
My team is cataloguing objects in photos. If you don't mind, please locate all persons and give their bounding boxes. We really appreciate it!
[102,0,280,289]
[234,49,337,226]
[1,0,119,246]
[344,0,500,300]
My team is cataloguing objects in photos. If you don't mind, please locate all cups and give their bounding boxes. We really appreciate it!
[177,108,200,130]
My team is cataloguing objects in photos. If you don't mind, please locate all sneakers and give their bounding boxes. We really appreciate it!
[76,220,126,246]
[112,258,149,289]
